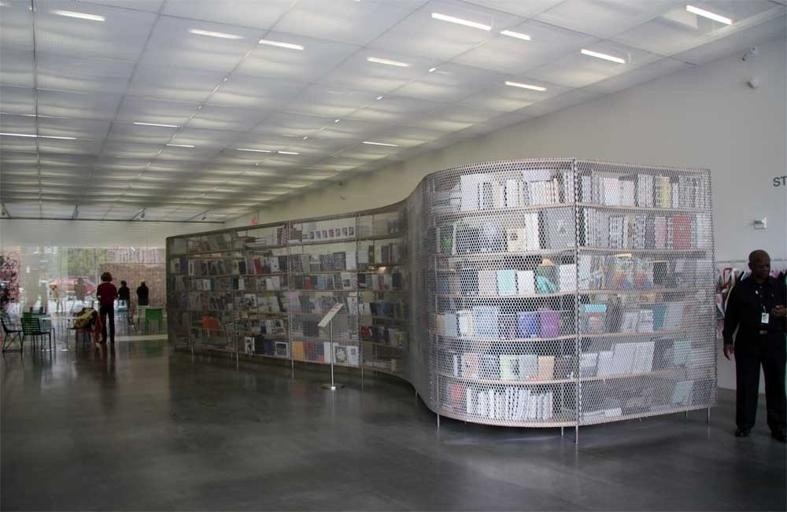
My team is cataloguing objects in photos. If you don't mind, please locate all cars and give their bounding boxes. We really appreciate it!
[36,275,97,301]
[0,280,24,305]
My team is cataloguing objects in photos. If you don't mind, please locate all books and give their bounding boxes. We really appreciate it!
[407,166,577,419]
[579,171,711,418]
[168,212,407,376]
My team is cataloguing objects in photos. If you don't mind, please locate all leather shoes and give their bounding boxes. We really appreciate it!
[735,426,751,436]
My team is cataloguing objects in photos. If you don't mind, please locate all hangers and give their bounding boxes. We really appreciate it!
[717,257,786,275]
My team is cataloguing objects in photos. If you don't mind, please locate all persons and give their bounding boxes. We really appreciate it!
[23,276,87,314]
[136,281,148,305]
[96,272,117,345]
[118,281,131,323]
[722,249,786,443]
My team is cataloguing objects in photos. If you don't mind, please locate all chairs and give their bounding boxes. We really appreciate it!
[0,302,164,355]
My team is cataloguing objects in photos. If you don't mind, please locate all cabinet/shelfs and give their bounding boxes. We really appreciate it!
[578,164,717,441]
[289,212,357,377]
[234,222,290,370]
[426,158,579,438]
[185,229,234,362]
[356,196,420,401]
[164,234,190,347]
